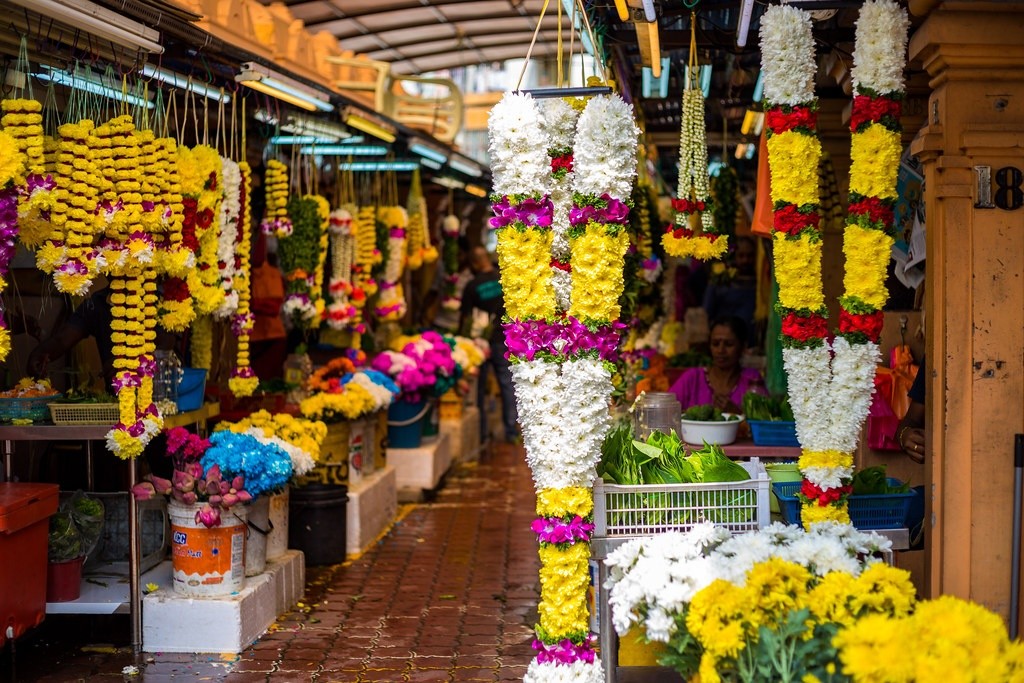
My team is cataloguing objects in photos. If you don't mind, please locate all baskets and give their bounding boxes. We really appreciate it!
[747,418,801,446]
[0,393,63,425]
[590,455,771,539]
[774,479,917,533]
[47,400,121,425]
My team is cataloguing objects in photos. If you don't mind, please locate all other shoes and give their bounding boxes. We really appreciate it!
[507,430,522,444]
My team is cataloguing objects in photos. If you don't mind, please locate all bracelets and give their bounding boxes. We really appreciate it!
[900,427,908,447]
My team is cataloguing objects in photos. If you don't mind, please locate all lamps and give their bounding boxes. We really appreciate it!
[681,56,713,99]
[612,0,658,24]
[752,63,766,103]
[736,0,754,47]
[268,134,365,147]
[9,0,165,55]
[339,104,398,143]
[133,61,231,105]
[740,105,764,138]
[338,160,420,172]
[407,132,449,164]
[734,138,757,161]
[250,102,350,139]
[27,61,157,112]
[446,152,482,180]
[298,142,391,157]
[641,50,674,99]
[235,60,335,115]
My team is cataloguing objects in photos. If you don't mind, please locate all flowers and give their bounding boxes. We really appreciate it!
[0,0,1024,683]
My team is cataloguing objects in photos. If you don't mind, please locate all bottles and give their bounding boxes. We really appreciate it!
[635,391,682,444]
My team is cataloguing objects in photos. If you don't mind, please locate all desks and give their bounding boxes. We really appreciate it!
[0,400,223,662]
[587,529,909,683]
[683,442,801,459]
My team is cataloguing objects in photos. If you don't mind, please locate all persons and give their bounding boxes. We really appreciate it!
[249,243,286,379]
[701,272,757,349]
[895,353,926,464]
[458,246,519,445]
[670,315,770,415]
[27,273,176,476]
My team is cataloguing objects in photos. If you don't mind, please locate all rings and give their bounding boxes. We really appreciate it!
[914,444,917,450]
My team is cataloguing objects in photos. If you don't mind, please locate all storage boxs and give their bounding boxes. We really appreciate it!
[0,481,64,645]
[0,392,64,419]
[439,406,482,460]
[46,401,119,425]
[346,465,400,556]
[142,571,278,654]
[387,432,455,492]
[773,476,919,529]
[268,548,306,624]
[593,456,773,535]
[749,420,800,447]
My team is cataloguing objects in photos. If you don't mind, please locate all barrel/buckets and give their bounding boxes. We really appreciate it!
[764,464,803,513]
[155,367,208,412]
[46,558,85,602]
[166,379,477,598]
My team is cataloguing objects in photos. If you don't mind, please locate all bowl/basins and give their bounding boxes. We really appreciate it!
[679,412,745,446]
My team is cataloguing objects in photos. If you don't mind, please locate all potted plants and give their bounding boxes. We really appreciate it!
[49,495,102,602]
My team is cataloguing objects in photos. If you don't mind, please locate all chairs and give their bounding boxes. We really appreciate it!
[324,53,465,143]
